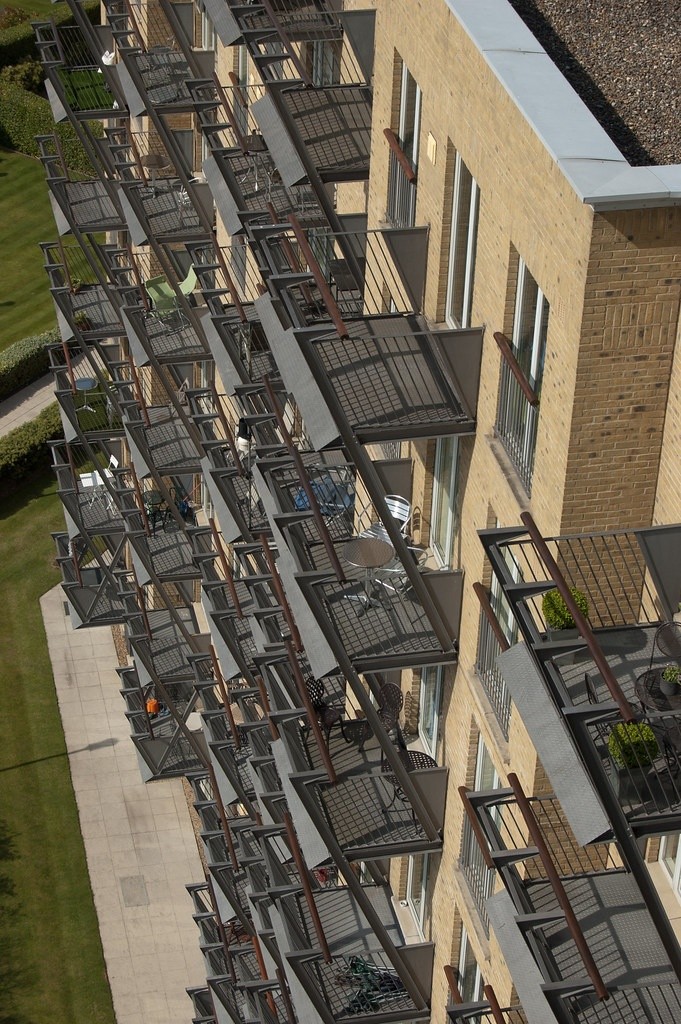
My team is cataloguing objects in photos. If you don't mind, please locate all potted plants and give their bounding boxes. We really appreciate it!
[607,721,658,807]
[542,586,590,665]
[74,311,91,331]
[63,275,82,296]
[659,665,681,695]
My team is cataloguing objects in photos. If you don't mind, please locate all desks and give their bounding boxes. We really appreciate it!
[74,378,98,412]
[327,257,365,301]
[381,751,438,834]
[239,135,270,191]
[142,490,166,538]
[79,472,108,508]
[634,667,681,728]
[147,45,175,78]
[141,154,170,198]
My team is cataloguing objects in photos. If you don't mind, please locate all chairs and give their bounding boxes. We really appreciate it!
[296,463,354,531]
[264,168,305,214]
[655,726,681,780]
[178,178,207,220]
[300,675,349,755]
[94,454,119,490]
[649,621,681,668]
[164,487,185,530]
[168,377,190,419]
[141,263,198,336]
[585,673,646,756]
[357,683,407,752]
[358,494,414,548]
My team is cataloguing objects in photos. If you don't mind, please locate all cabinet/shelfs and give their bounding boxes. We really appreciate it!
[342,538,395,617]
